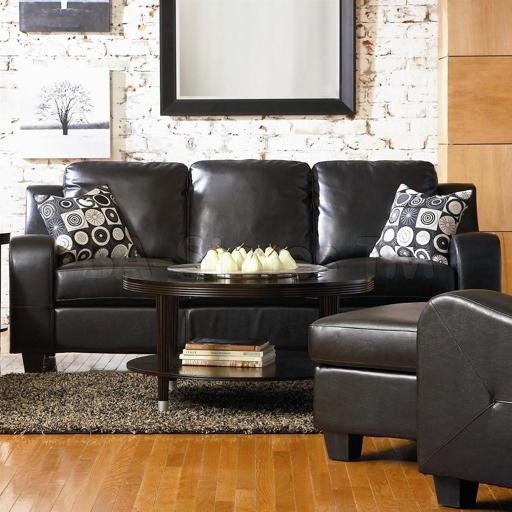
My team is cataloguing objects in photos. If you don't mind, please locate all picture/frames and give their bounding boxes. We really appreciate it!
[160,0,356,115]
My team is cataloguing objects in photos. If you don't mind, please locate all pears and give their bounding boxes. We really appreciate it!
[200,242,296,273]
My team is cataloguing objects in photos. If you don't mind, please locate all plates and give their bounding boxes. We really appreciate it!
[167,263,328,278]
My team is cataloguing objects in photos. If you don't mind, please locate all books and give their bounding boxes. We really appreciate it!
[177,334,277,368]
[178,364,277,380]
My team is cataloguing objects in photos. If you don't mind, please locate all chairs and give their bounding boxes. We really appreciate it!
[416,289,512,508]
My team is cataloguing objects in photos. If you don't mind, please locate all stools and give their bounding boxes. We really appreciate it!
[307,301,429,460]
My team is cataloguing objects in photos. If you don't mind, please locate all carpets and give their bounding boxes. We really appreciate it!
[0,370,318,435]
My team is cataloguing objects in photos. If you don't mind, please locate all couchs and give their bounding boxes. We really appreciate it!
[9,159,501,374]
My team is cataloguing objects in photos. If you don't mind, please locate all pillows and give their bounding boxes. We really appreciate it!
[34,184,140,264]
[368,183,474,266]
